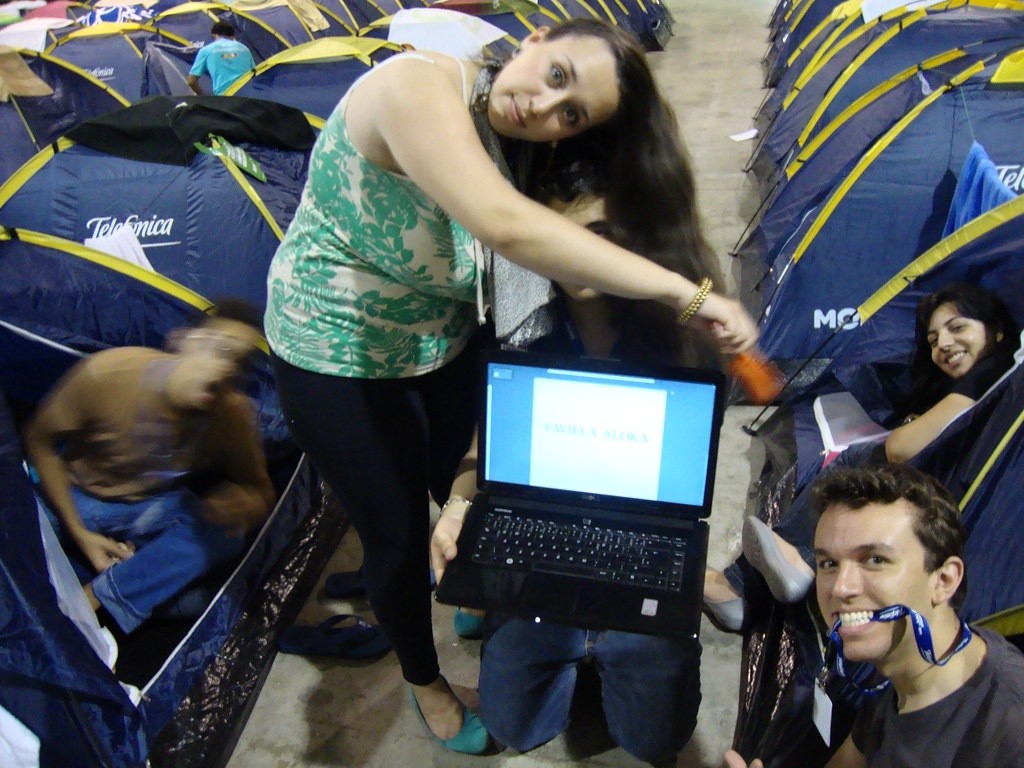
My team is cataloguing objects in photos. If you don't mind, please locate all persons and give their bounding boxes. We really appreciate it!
[22,300,275,635]
[703,282,1019,631]
[432,184,732,767]
[261,16,758,752]
[725,457,1023,767]
[187,20,256,96]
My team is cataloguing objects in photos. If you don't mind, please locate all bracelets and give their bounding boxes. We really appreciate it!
[675,277,714,325]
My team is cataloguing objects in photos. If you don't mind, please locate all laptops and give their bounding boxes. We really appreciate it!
[434,346,729,640]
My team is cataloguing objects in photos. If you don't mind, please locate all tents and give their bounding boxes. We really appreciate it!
[258,2,676,95]
[148,1,356,65]
[0,1,186,94]
[736,410,1023,651]
[0,96,343,768]
[730,0,1023,455]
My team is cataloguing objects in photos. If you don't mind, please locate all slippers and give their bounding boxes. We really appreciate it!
[279,615,391,662]
[326,558,436,599]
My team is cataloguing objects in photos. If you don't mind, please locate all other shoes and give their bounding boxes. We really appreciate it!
[742,515,816,604]
[453,606,490,641]
[409,673,488,754]
[704,565,743,633]
